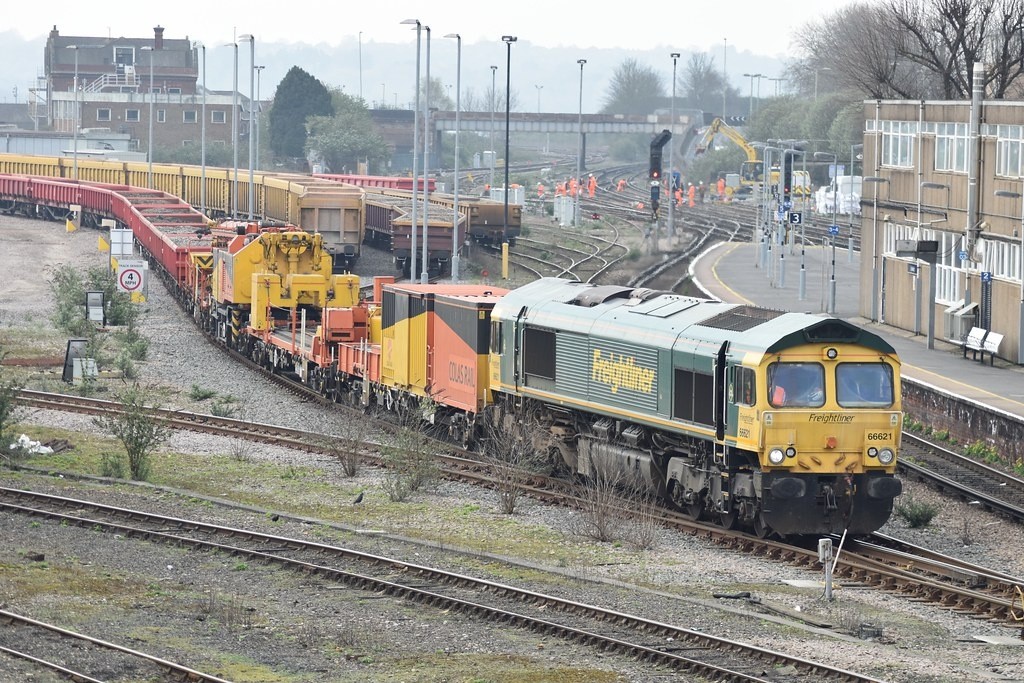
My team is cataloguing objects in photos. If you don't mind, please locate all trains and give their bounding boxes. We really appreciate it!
[364,192,466,279]
[311,174,437,193]
[359,186,522,247]
[0,173,903,549]
[0,152,365,272]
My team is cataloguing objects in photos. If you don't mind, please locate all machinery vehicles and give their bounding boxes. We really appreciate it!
[695,117,810,210]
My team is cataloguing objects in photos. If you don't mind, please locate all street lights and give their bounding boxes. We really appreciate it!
[224,34,265,221]
[491,66,498,191]
[443,33,461,281]
[412,26,430,285]
[574,60,587,225]
[400,18,421,285]
[501,35,517,279]
[668,52,680,243]
[745,139,863,318]
[192,45,205,216]
[142,46,154,189]
[66,45,78,179]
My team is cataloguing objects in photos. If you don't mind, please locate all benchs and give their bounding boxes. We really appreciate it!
[964,332,1004,366]
[948,327,987,360]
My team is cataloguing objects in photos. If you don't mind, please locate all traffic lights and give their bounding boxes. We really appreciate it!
[592,213,599,220]
